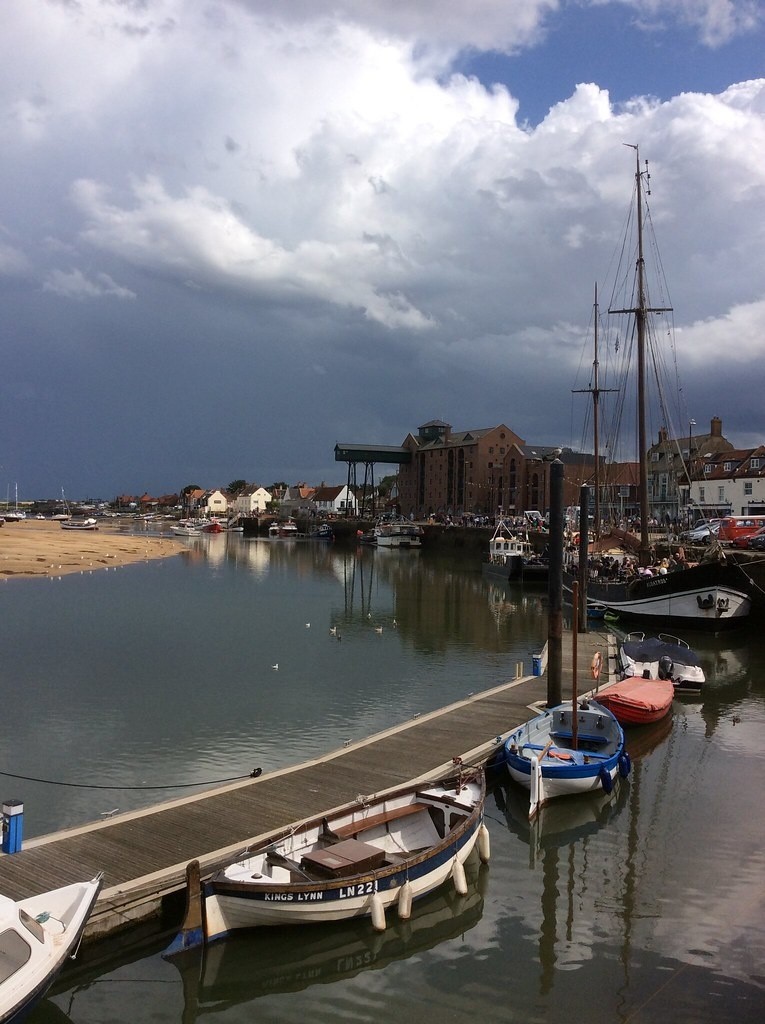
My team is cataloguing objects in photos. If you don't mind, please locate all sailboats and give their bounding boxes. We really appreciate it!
[562,142,753,626]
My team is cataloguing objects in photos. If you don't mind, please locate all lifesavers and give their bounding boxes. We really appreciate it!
[591,652,604,678]
[488,553,493,561]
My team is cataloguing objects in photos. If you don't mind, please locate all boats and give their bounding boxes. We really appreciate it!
[0,481,422,548]
[620,633,704,695]
[586,604,608,618]
[590,677,675,726]
[164,766,495,958]
[0,869,106,1024]
[479,518,548,584]
[508,701,628,824]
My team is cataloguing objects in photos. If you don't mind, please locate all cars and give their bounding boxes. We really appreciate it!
[674,516,765,551]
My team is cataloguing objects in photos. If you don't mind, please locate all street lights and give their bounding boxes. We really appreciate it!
[688,419,697,502]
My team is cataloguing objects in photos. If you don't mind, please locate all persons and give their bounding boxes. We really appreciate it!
[408,498,727,583]
